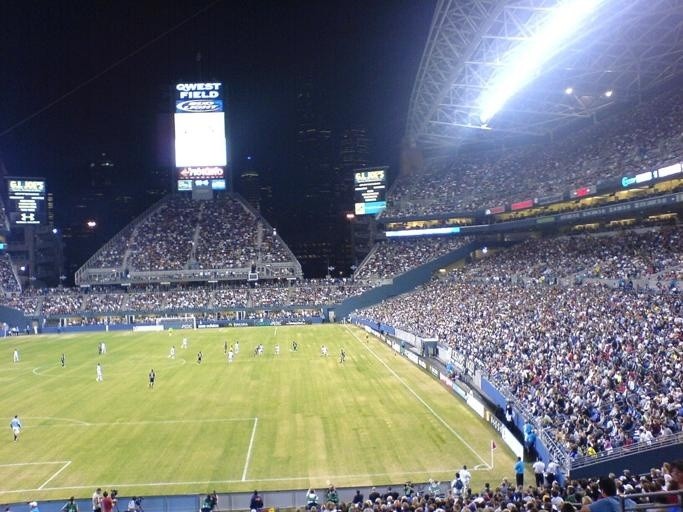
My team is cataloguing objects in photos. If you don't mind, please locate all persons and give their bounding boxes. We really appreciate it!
[200,495,216,512]
[9,416,21,442]
[27,501,39,512]
[92,488,117,512]
[0,237,22,279]
[0,320,31,336]
[127,496,138,512]
[0,278,293,389]
[79,191,292,279]
[210,491,218,512]
[249,490,263,512]
[293,89,683,512]
[60,496,79,512]
